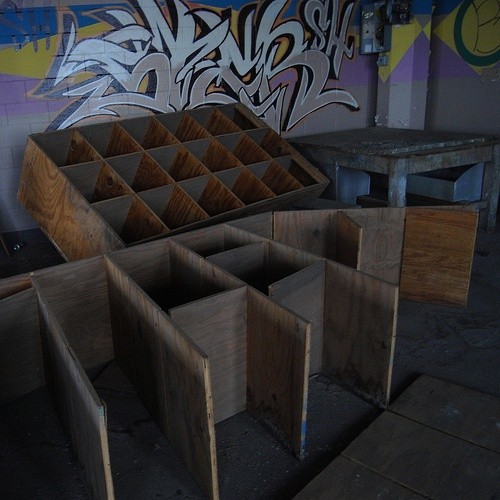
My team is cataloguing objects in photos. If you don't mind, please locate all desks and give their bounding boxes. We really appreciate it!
[283,126,500,258]
